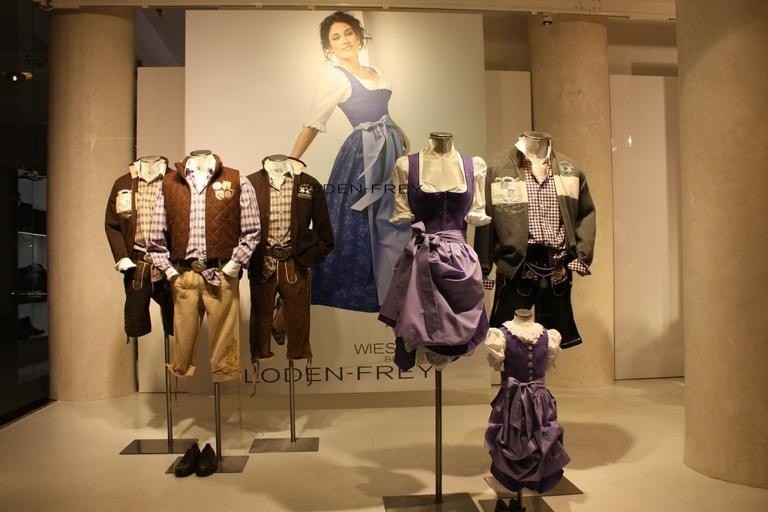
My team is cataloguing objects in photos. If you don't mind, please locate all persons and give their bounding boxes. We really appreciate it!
[389,132,493,373]
[105,154,174,337]
[245,154,334,359]
[290,10,411,314]
[473,130,596,350]
[148,150,262,383]
[485,309,571,494]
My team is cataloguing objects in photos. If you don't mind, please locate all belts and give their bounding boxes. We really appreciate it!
[265,247,292,261]
[179,258,227,273]
[133,250,153,264]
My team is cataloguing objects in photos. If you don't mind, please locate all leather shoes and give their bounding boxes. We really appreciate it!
[198,443,215,476]
[175,443,200,477]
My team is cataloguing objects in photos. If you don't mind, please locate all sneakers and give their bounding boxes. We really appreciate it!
[18,317,45,337]
